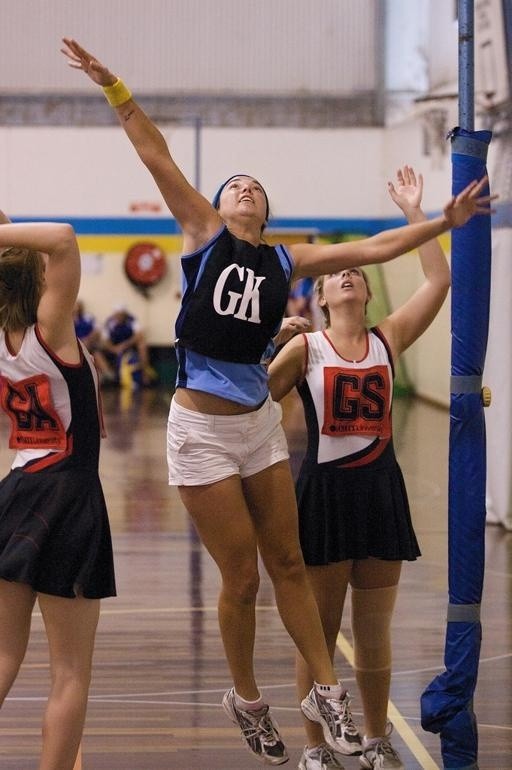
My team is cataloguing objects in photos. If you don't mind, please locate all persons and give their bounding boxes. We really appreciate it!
[1,210,121,767]
[94,302,160,390]
[73,300,114,383]
[262,165,450,770]
[61,37,499,764]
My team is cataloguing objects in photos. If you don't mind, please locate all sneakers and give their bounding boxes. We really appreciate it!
[297,682,405,769]
[221,687,289,765]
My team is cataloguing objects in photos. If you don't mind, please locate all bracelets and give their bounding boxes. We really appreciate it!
[103,76,133,107]
